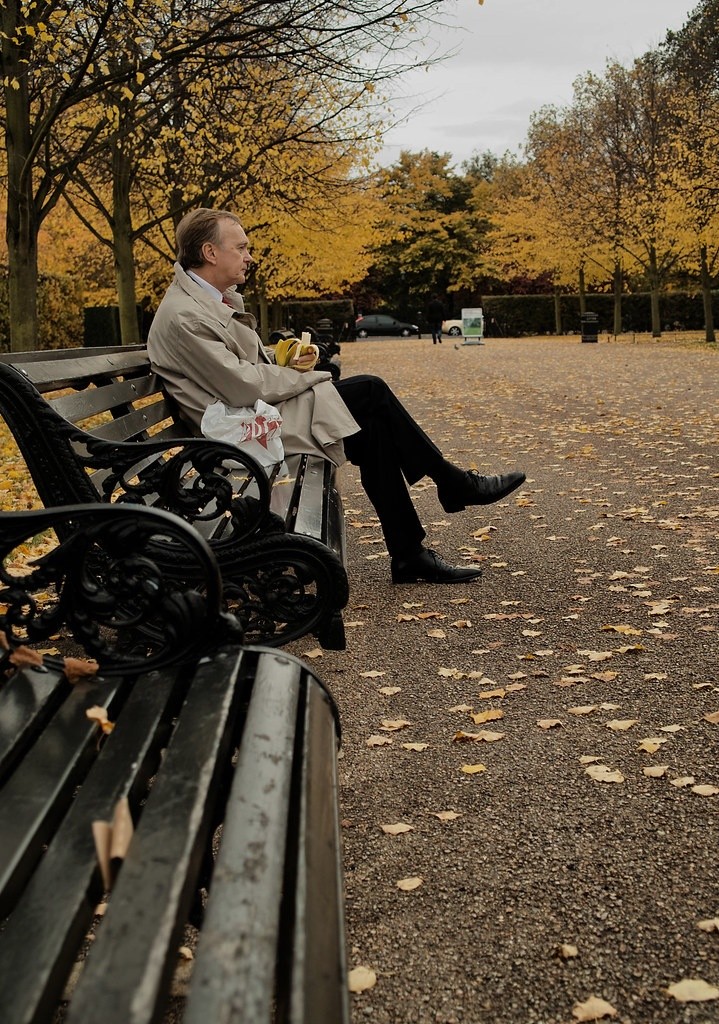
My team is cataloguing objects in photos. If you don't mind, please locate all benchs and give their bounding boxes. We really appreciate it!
[0,503,351,1024]
[267,330,342,381]
[0,342,350,651]
[305,326,334,343]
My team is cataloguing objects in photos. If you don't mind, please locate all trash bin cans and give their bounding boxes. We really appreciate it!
[317,318,334,343]
[579,312,598,343]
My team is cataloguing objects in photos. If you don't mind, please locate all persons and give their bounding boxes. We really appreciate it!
[425,293,446,344]
[147,208,526,583]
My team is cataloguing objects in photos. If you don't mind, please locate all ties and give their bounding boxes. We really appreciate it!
[222,296,233,308]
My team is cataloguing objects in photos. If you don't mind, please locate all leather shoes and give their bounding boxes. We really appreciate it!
[391,547,482,584]
[436,469,527,513]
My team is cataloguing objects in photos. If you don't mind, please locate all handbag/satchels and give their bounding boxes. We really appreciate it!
[202,398,285,470]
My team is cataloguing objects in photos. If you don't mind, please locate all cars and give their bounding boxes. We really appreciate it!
[355,314,419,339]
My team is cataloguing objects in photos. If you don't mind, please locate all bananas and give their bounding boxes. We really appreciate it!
[274,331,319,371]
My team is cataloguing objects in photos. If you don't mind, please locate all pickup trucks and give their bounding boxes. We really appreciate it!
[442,317,463,336]
